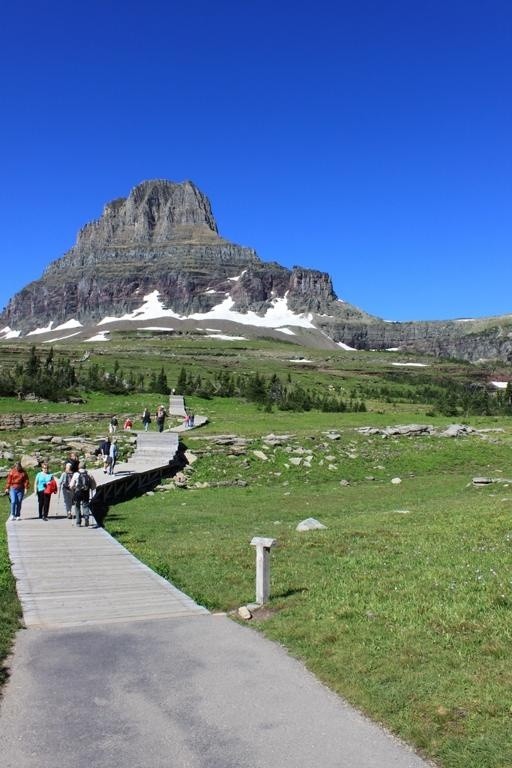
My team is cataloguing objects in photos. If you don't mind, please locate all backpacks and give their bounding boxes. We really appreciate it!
[75,472,90,490]
[63,472,74,490]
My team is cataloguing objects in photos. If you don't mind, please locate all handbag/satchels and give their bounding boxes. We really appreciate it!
[74,490,89,501]
[106,457,112,463]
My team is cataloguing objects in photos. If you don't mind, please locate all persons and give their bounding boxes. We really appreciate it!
[185,413,194,428]
[4,405,169,527]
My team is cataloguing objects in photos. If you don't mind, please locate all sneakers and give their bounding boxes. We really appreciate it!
[68,513,76,518]
[104,470,113,475]
[39,515,46,519]
[76,517,89,527]
[12,515,21,520]
